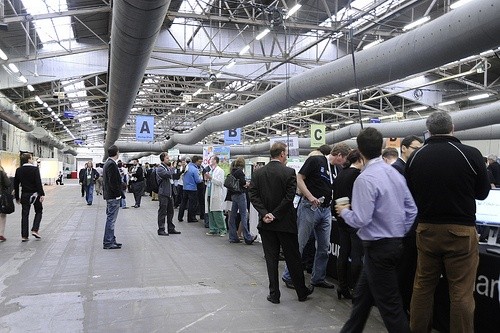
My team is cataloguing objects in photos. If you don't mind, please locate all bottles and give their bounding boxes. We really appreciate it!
[311,196,325,211]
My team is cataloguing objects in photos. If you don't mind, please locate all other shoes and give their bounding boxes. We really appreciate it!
[131,204,140,208]
[32,230,41,238]
[22,237,30,241]
[249,234,257,245]
[0,194,15,214]
[299,284,314,301]
[168,228,181,234]
[267,295,280,303]
[0,235,7,240]
[158,230,169,236]
[229,238,243,243]
[310,279,334,288]
[220,234,226,237]
[282,277,296,289]
[206,230,220,235]
[103,241,122,249]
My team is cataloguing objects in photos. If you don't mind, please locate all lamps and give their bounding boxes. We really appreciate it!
[226,60,236,69]
[10,64,28,84]
[27,84,34,92]
[240,44,251,56]
[35,95,75,140]
[469,93,489,101]
[255,28,270,41]
[413,106,427,112]
[0,49,9,61]
[439,100,456,107]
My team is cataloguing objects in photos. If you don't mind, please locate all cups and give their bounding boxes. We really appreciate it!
[206,172,210,180]
[335,197,349,210]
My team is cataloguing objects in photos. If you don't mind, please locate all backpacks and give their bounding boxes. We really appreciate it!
[148,166,166,193]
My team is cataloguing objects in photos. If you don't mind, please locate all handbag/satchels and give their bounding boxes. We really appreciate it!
[224,168,241,193]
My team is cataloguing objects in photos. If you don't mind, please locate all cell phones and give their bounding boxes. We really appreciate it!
[424,131,430,140]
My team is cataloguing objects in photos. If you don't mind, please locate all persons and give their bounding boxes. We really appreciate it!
[156,152,182,235]
[103,145,126,249]
[335,128,418,333]
[55,136,500,301]
[250,143,314,304]
[405,112,491,333]
[282,143,352,289]
[0,166,13,241]
[15,153,46,241]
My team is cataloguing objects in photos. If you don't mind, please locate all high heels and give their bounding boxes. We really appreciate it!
[336,287,352,299]
